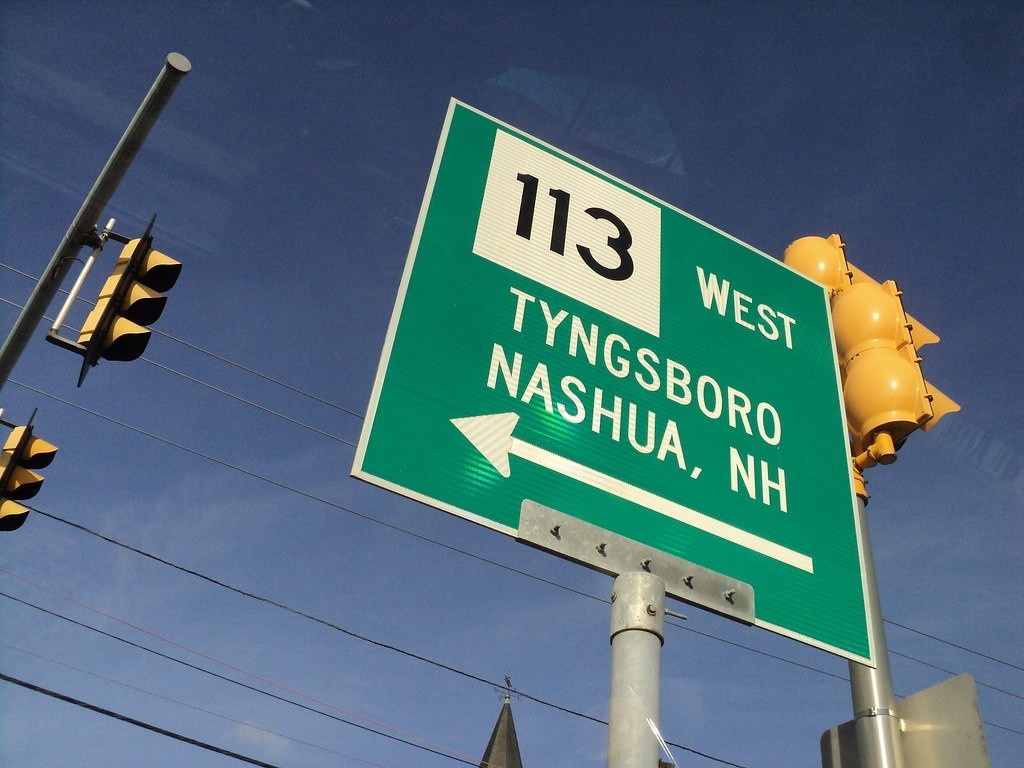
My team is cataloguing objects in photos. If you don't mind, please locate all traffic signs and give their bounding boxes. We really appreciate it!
[350,96,878,670]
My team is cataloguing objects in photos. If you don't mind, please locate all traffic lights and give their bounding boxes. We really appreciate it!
[74,211,185,386]
[775,233,961,476]
[0,406,58,532]
